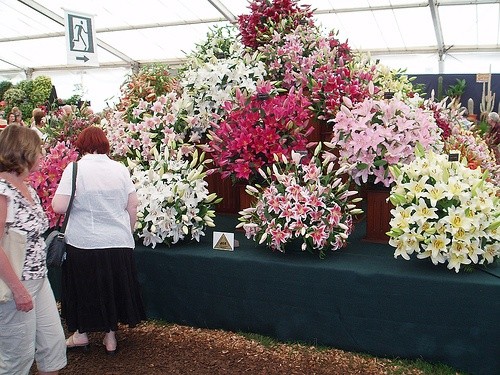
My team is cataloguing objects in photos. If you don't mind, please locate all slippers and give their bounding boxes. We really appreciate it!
[66,335,88,348]
[103,336,117,352]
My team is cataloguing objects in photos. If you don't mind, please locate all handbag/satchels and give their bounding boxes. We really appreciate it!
[44,231,65,267]
[0,185,27,303]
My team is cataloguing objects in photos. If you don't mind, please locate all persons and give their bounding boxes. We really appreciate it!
[52,126,137,352]
[486,112,500,145]
[6,107,47,140]
[0,126,67,375]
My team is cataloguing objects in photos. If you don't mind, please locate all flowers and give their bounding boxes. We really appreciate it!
[0,0,500,273]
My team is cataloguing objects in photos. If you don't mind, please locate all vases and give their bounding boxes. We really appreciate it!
[236,183,270,232]
[322,132,366,196]
[194,137,247,215]
[367,185,396,244]
[302,90,333,159]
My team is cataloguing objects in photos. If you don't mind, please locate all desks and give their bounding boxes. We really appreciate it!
[120,214,500,375]
